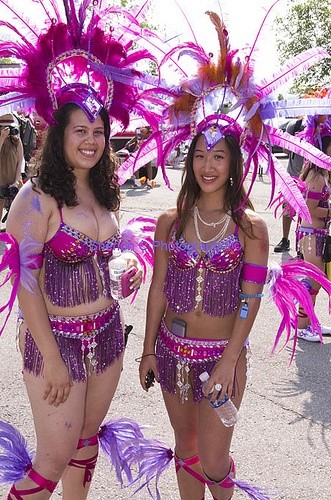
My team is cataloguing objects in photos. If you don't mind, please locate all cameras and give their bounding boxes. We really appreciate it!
[0,184,19,200]
[4,125,19,135]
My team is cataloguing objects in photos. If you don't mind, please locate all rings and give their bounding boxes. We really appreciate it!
[215,384,222,391]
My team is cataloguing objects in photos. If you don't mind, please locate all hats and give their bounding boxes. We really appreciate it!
[0,113,21,127]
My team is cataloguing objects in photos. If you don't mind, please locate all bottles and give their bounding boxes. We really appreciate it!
[108,248,127,301]
[199,371,240,427]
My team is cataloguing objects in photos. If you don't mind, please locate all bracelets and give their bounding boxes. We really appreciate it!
[136,353,158,362]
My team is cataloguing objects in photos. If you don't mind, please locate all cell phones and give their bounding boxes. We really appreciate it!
[171,318,187,338]
[146,371,154,388]
[120,266,137,298]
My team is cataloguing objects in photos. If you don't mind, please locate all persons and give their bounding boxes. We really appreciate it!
[274,117,331,341]
[6,102,142,500]
[139,126,269,500]
[0,109,46,222]
[124,126,156,190]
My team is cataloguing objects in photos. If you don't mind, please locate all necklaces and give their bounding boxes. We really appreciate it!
[193,207,232,252]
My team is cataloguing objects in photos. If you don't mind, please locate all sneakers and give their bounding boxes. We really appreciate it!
[308,324,331,335]
[296,328,320,342]
[274,238,290,252]
